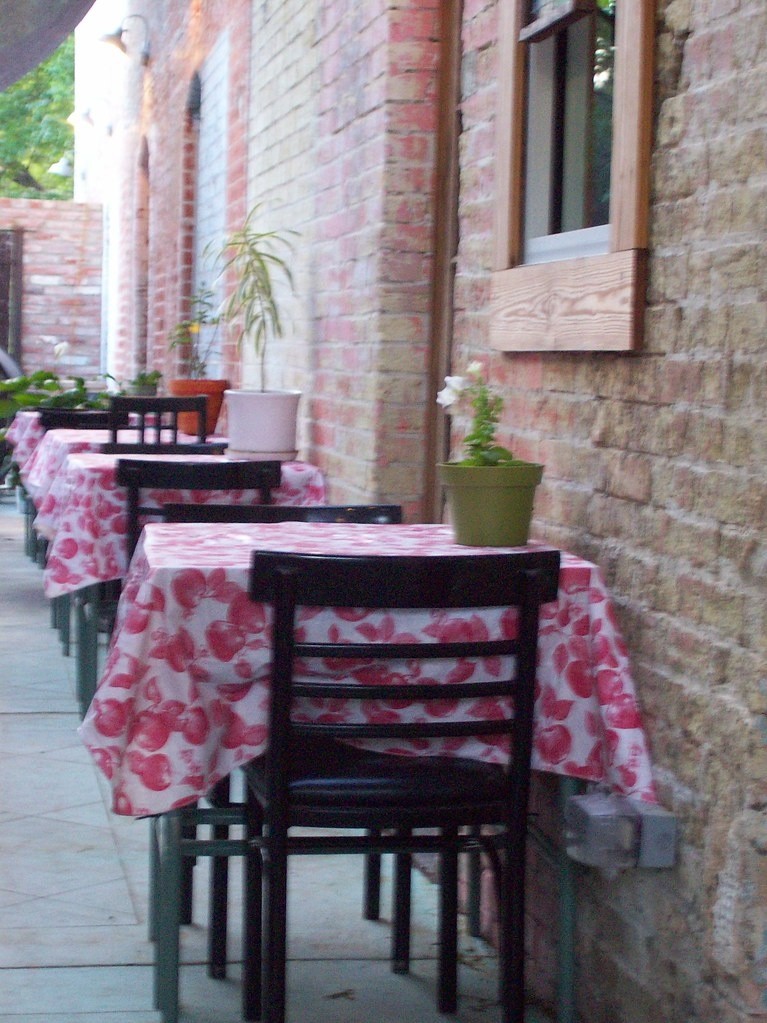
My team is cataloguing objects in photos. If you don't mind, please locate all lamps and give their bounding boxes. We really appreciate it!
[67,108,111,142]
[91,14,149,67]
[48,157,91,184]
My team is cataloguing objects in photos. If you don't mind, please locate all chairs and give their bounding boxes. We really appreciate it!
[37,393,561,1022]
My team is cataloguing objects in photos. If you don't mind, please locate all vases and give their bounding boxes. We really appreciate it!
[444,460,544,546]
[167,378,230,436]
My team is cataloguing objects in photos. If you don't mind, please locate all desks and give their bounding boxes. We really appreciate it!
[5,409,660,1022]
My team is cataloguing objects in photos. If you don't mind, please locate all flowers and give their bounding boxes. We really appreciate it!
[167,281,224,380]
[436,359,529,466]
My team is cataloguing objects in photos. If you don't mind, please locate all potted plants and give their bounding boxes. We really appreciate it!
[127,371,162,396]
[200,202,304,460]
